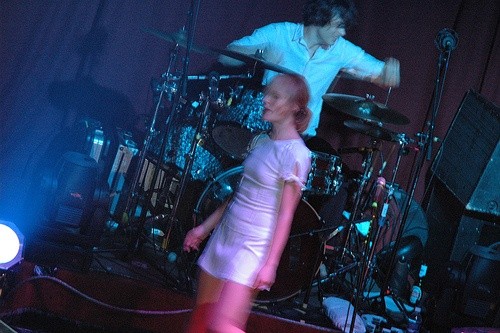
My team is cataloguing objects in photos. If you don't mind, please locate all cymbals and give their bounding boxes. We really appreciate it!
[141,26,210,55]
[204,44,305,79]
[344,120,398,142]
[321,93,410,126]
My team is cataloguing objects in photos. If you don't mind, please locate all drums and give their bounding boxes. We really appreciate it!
[193,166,326,303]
[301,150,343,196]
[209,83,273,161]
[146,95,226,190]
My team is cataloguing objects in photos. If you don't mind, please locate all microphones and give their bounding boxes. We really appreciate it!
[436,28,458,51]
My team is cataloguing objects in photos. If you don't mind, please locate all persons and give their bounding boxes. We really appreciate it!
[182,73,311,333]
[216,0,400,181]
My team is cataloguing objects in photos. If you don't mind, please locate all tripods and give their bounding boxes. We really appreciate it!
[316,49,453,333]
[89,43,216,294]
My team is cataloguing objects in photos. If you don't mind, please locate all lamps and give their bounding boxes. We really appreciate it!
[23,151,110,273]
[0,220,25,270]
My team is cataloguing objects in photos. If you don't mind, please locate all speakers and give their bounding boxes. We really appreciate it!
[395,172,500,333]
[429,86,500,218]
